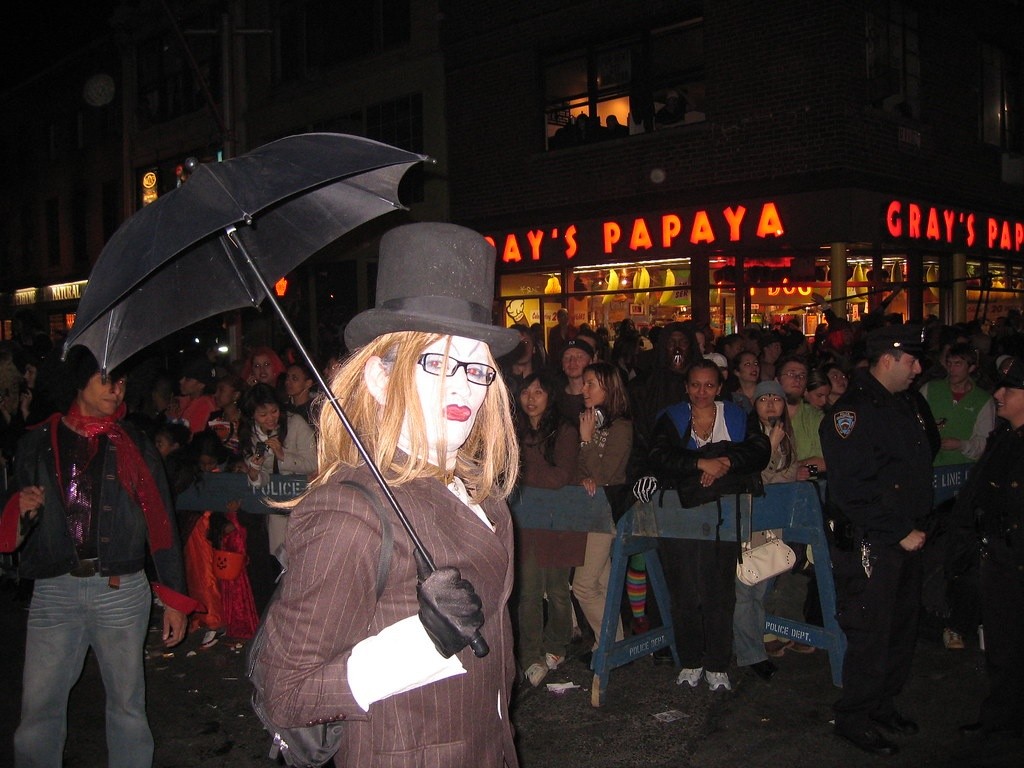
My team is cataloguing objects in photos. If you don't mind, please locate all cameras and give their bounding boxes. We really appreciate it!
[805,465,818,475]
[582,407,606,429]
[256,442,266,461]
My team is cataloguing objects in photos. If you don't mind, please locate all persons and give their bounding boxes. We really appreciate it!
[818,326,942,756]
[494,309,1024,692]
[0,343,344,657]
[247,222,520,768]
[936,357,1024,734]
[0,346,207,768]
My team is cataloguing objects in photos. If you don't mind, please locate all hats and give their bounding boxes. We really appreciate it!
[996,360,1024,389]
[344,222,521,359]
[867,326,924,354]
[754,381,788,403]
[563,339,594,357]
[996,355,1013,370]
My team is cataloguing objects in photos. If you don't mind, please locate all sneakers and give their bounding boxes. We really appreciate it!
[676,667,703,688]
[943,628,965,649]
[833,699,918,758]
[546,652,565,669]
[704,670,731,691]
[524,664,549,688]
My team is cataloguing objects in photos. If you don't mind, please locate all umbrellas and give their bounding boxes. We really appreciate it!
[64,132,491,659]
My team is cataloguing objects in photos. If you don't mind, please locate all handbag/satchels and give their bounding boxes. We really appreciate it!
[736,530,796,587]
[212,548,244,581]
[679,440,765,509]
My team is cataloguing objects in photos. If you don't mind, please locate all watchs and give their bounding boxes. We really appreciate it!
[804,463,818,476]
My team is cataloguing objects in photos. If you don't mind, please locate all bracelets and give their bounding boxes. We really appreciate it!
[580,441,590,448]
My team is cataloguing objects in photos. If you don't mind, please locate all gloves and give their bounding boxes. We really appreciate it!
[633,476,659,503]
[413,548,485,659]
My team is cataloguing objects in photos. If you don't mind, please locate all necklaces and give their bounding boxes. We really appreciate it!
[691,404,716,440]
[691,402,716,449]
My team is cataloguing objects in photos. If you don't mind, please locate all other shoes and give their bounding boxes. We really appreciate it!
[751,632,816,683]
[579,650,593,663]
[959,721,985,736]
[196,631,246,653]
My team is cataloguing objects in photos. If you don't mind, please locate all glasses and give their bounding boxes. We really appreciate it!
[782,371,806,379]
[384,352,496,386]
